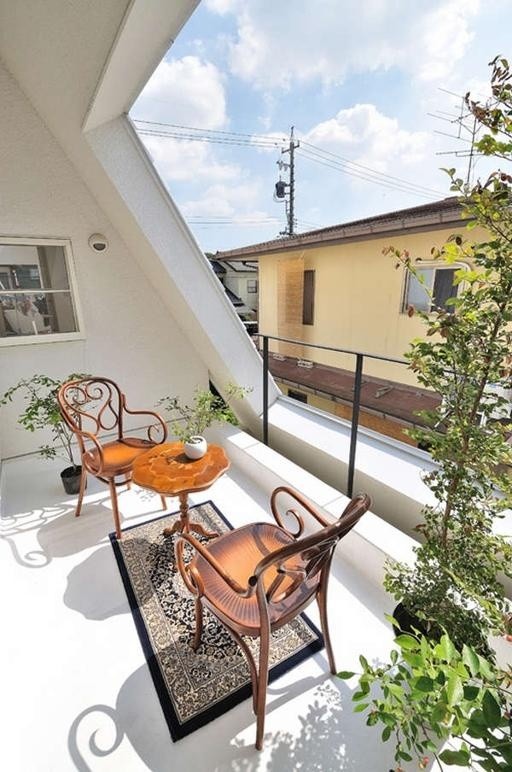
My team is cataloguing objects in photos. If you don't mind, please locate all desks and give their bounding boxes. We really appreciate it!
[132,439,231,539]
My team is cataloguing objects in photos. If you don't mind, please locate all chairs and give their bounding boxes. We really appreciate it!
[58,377,167,539]
[176,487,372,752]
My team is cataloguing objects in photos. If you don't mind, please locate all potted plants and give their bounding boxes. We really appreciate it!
[383,55,512,664]
[0,371,92,494]
[154,383,255,461]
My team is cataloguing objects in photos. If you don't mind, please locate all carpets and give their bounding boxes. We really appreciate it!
[110,500,327,742]
